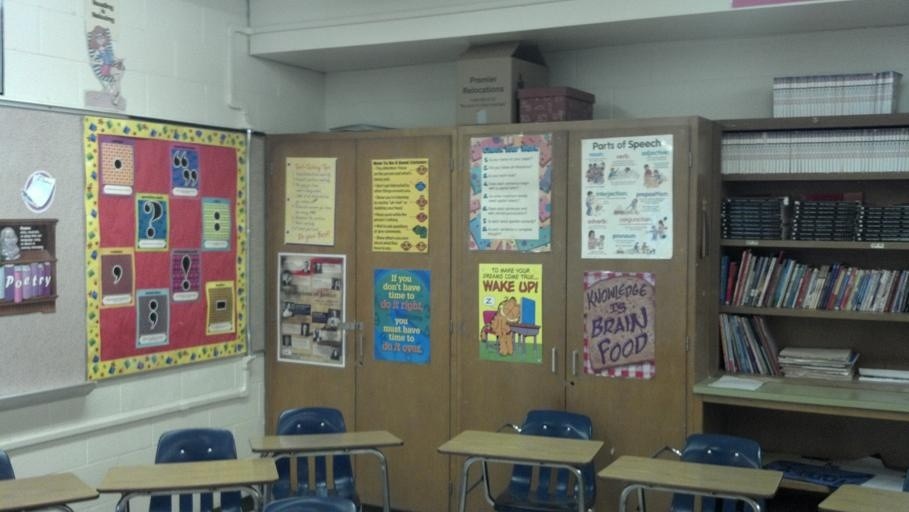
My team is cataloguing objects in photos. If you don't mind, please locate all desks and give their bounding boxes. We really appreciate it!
[818,484,909,512]
[96,457,280,512]
[598,456,783,512]
[250,430,404,512]
[438,429,605,512]
[0,472,99,512]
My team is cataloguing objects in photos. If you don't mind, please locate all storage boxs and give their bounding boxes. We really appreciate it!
[454,41,547,124]
[517,86,595,122]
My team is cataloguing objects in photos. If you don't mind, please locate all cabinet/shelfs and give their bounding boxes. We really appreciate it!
[693,113,909,496]
[265,131,451,512]
[453,116,721,512]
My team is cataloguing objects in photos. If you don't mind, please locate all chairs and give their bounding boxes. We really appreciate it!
[149,427,243,512]
[492,409,592,512]
[672,434,762,512]
[264,495,356,512]
[0,449,15,480]
[263,406,358,512]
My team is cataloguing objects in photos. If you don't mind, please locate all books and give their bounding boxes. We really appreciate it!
[720,129,909,174]
[721,192,909,242]
[719,314,909,380]
[719,251,909,314]
[773,73,904,118]
